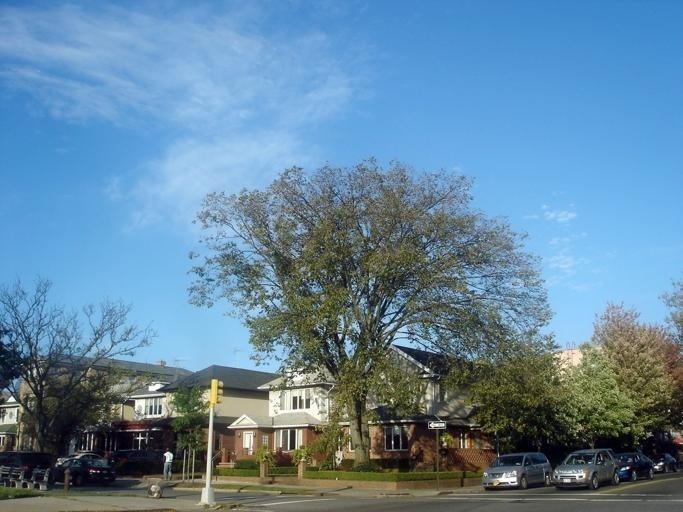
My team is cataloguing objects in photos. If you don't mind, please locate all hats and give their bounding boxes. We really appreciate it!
[166,448,170,450]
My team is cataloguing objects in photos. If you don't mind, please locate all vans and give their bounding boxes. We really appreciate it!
[482,452,553,491]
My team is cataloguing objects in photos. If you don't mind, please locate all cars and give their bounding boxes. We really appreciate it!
[615,453,655,483]
[112,448,165,476]
[50,457,116,488]
[647,453,678,474]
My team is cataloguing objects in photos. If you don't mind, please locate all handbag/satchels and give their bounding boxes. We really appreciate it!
[161,455,166,463]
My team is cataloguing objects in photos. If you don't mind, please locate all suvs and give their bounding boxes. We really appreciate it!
[551,448,622,490]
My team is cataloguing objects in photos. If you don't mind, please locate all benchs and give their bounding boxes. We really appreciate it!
[0,464,51,491]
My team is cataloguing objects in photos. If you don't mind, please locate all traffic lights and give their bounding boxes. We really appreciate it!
[210,378,224,404]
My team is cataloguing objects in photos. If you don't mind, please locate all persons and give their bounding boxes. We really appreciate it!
[162,448,174,482]
